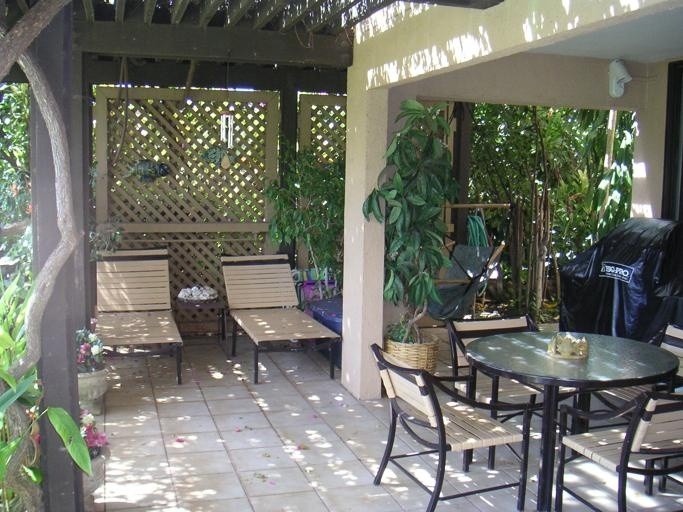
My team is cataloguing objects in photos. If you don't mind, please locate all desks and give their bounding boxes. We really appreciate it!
[464,331,679,512]
[172,296,224,362]
[173,300,225,344]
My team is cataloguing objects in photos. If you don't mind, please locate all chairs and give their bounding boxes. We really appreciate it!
[591,297,683,494]
[371,343,533,511]
[447,314,579,463]
[221,254,342,384]
[555,391,683,512]
[94,249,183,384]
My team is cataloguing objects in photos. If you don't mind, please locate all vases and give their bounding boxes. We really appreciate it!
[77,364,110,416]
[82,456,106,512]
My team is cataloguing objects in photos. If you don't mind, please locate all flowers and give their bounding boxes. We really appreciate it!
[78,411,108,459]
[75,324,103,373]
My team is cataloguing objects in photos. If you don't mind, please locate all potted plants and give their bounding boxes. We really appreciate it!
[361,98,457,375]
